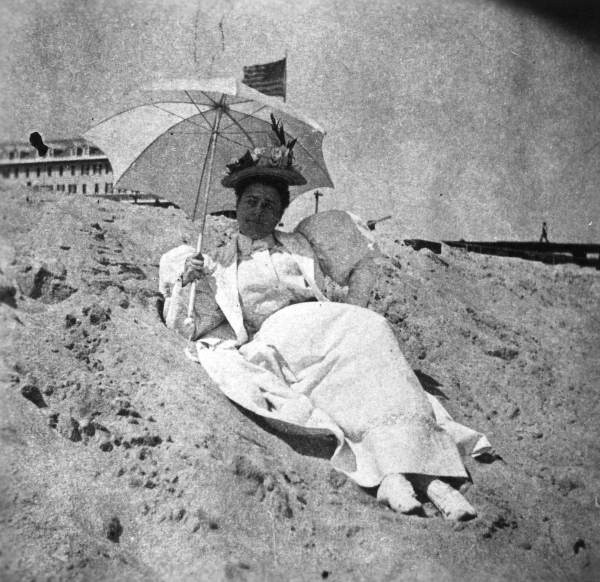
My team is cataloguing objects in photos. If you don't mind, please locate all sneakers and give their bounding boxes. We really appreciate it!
[377,477,421,512]
[427,479,476,522]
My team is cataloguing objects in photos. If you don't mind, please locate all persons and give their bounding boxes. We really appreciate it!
[163,113,492,523]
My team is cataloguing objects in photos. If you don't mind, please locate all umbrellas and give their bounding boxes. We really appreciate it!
[80,77,335,328]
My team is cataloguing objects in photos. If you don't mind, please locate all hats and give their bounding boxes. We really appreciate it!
[222,113,306,187]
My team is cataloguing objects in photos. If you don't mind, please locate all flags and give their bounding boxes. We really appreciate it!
[240,59,286,99]
